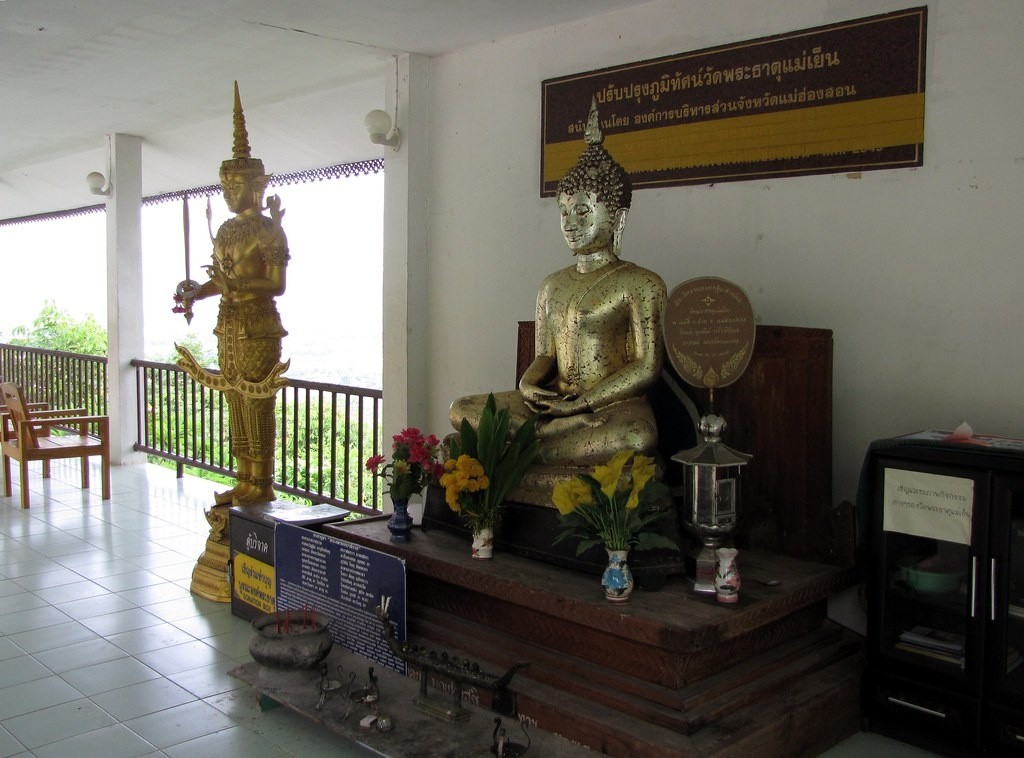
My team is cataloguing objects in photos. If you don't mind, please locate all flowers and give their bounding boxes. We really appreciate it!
[438,392,543,532]
[549,446,678,555]
[365,428,444,498]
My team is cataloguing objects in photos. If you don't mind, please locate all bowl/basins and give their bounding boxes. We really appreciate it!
[899,557,966,594]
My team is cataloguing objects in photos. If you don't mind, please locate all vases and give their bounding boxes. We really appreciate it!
[600,547,638,601]
[472,527,495,559]
[386,495,414,543]
[715,547,741,603]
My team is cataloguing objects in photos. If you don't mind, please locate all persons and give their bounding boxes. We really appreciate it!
[177,81,290,508]
[442,94,667,466]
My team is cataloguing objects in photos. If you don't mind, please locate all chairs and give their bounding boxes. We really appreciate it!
[0,380,111,509]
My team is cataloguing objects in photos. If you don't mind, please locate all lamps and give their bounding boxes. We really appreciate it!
[671,415,755,594]
[363,110,402,153]
[86,171,113,199]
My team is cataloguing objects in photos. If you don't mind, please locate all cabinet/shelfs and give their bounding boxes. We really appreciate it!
[859,442,1024,758]
